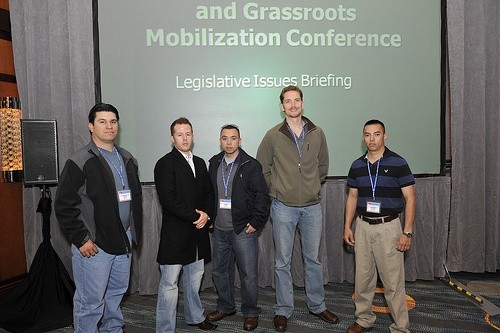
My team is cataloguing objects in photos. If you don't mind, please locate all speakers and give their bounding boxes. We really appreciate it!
[20,119,60,186]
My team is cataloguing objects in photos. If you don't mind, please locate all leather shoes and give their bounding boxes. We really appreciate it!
[206,310,236,321]
[272,315,287,332]
[243,316,258,331]
[187,320,218,330]
[346,322,366,333]
[309,309,339,324]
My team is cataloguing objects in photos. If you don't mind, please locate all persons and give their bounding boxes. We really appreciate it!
[154,116,218,333]
[54,102,143,333]
[207,123,272,331]
[342,119,417,333]
[255,85,339,333]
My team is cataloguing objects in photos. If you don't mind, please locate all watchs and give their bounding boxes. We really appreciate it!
[402,232,414,238]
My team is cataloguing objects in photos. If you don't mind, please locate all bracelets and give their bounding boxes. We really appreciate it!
[207,216,211,220]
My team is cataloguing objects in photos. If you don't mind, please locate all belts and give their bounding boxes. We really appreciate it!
[359,214,399,224]
